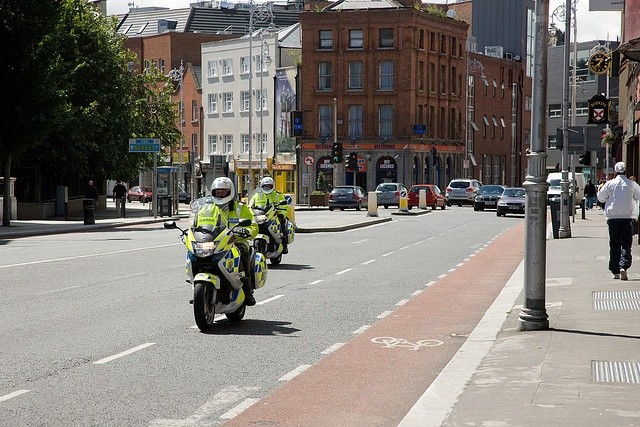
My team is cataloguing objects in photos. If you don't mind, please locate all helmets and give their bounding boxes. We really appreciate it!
[211,177,235,206]
[260,177,275,195]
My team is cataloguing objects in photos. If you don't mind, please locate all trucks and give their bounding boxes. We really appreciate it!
[546,171,586,208]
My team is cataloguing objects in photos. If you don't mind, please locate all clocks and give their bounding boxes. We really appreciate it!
[588,52,610,74]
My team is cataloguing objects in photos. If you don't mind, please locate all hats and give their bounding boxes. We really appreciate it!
[613,161,626,174]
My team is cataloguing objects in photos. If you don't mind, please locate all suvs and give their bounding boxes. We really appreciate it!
[375,182,409,208]
[445,178,483,208]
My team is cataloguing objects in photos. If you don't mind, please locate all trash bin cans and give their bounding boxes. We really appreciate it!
[160,197,171,218]
[550,197,571,239]
[83,199,95,225]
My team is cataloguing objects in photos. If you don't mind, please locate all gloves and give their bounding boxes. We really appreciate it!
[238,228,249,237]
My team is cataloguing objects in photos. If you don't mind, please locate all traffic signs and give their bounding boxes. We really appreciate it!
[129,138,160,152]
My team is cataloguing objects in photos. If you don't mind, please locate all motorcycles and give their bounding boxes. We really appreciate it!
[237,195,296,264]
[163,197,270,332]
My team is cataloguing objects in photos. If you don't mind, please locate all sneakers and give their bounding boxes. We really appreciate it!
[282,242,288,254]
[619,268,628,281]
[612,273,620,280]
[242,286,255,306]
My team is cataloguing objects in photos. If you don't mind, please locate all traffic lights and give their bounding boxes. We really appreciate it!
[345,151,358,170]
[332,142,342,162]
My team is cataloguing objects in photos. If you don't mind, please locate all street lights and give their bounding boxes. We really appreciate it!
[163,59,183,183]
[548,0,576,216]
[247,6,280,204]
[463,49,486,178]
[261,39,277,186]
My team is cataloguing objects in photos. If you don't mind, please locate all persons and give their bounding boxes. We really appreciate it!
[596,160,639,278]
[179,175,260,306]
[111,178,127,218]
[582,177,595,210]
[246,175,289,254]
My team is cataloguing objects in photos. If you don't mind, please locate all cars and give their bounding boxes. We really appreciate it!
[496,186,525,216]
[406,183,448,209]
[327,185,367,210]
[474,184,507,210]
[127,185,152,202]
[179,186,190,203]
